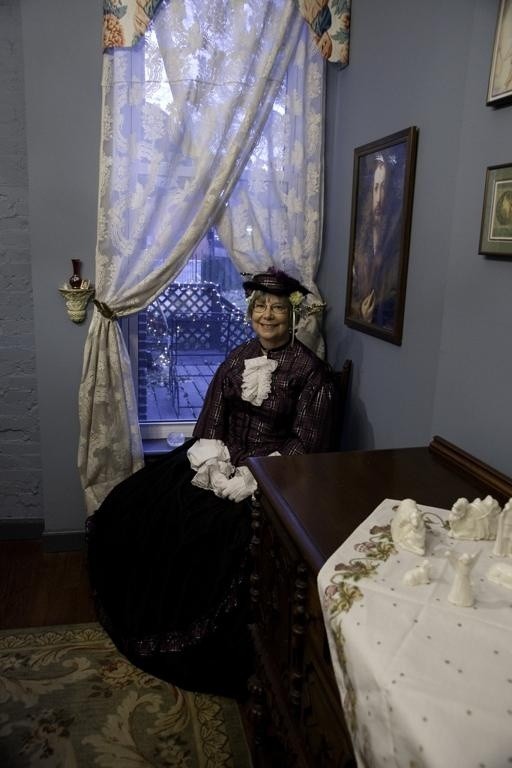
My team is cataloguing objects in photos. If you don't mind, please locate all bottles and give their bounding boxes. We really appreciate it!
[166,431,185,447]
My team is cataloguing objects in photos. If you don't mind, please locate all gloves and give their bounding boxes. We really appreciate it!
[209,467,250,503]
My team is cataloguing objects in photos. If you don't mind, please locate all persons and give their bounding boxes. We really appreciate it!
[79,265,340,702]
[348,153,403,334]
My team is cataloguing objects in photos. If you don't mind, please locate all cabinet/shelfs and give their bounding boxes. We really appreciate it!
[247,436,510,768]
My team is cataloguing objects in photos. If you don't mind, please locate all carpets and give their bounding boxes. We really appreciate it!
[2,622,257,766]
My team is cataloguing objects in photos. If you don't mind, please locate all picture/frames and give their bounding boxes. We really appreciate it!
[343,125,418,349]
[476,1,511,256]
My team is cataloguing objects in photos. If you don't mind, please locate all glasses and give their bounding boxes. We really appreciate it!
[252,303,289,314]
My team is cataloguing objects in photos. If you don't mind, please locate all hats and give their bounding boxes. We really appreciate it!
[242,272,298,297]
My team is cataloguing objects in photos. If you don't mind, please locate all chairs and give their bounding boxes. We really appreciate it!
[326,360,357,451]
